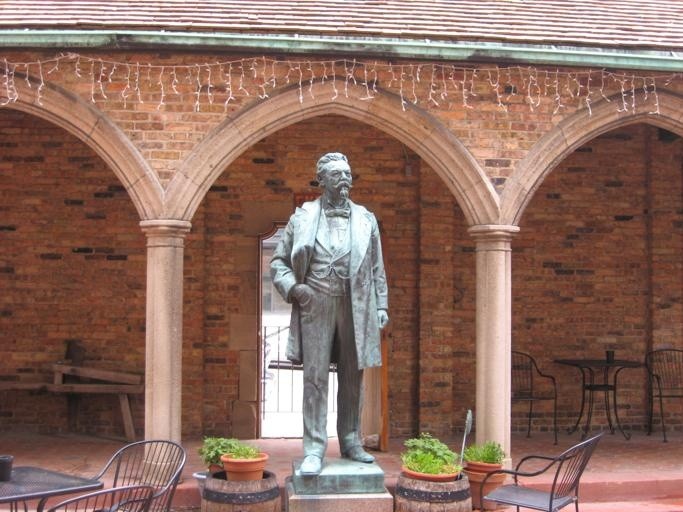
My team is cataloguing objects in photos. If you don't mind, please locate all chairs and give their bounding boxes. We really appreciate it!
[645,349,683,443]
[510,350,558,446]
[478,430,605,512]
[37,438,186,511]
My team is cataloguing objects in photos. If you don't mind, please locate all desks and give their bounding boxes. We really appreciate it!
[0,466,103,511]
[553,358,644,441]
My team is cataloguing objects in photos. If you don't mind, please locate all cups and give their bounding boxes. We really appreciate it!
[0,455,15,483]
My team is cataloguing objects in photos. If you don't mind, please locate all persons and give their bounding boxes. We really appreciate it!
[270,152,389,478]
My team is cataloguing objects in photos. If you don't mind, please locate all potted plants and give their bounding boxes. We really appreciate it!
[391,430,506,512]
[193,435,283,512]
